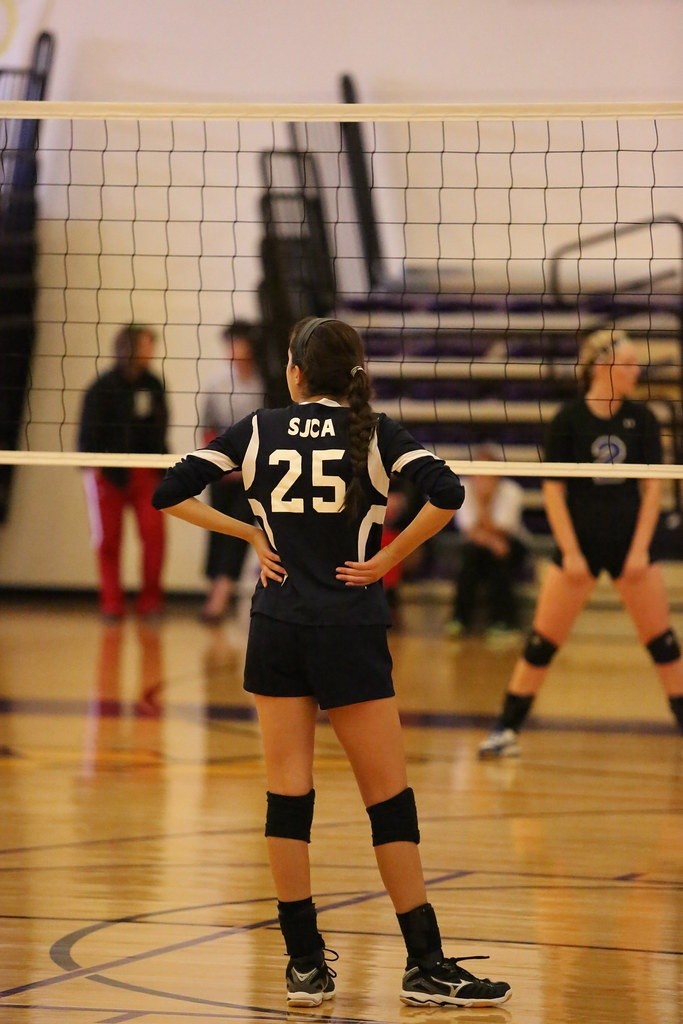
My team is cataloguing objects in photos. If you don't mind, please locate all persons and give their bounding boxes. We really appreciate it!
[151,317,512,1008]
[75,326,177,624]
[444,443,531,638]
[478,330,683,760]
[197,320,276,626]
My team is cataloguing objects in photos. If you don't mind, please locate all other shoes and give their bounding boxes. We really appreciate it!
[446,620,467,640]
[479,729,523,758]
[487,630,522,648]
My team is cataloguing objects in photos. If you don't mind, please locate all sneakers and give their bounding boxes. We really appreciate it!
[284,949,339,1008]
[400,955,512,1008]
